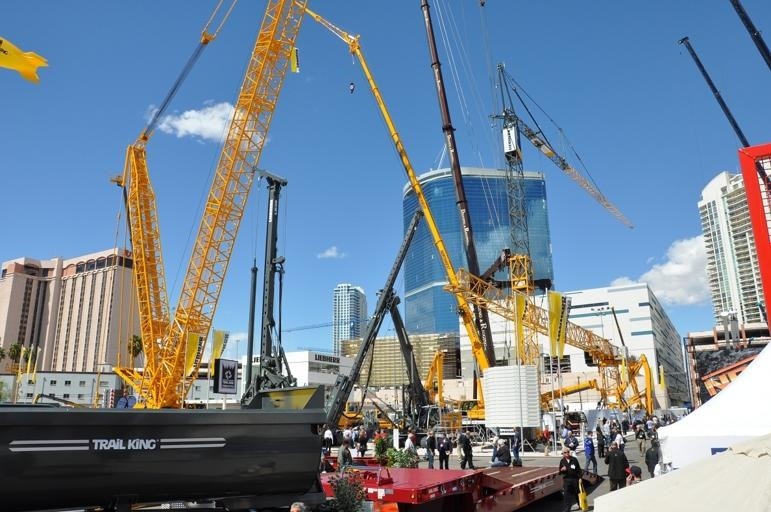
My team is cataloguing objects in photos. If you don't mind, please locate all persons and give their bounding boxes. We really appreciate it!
[544,412,679,512]
[490,428,520,467]
[323,425,389,473]
[406,429,475,470]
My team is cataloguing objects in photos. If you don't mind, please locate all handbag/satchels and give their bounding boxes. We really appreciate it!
[578,492,588,512]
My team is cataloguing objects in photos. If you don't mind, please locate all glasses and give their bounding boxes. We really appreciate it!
[564,451,569,454]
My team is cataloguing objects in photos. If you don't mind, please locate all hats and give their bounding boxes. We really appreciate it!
[625,465,641,476]
